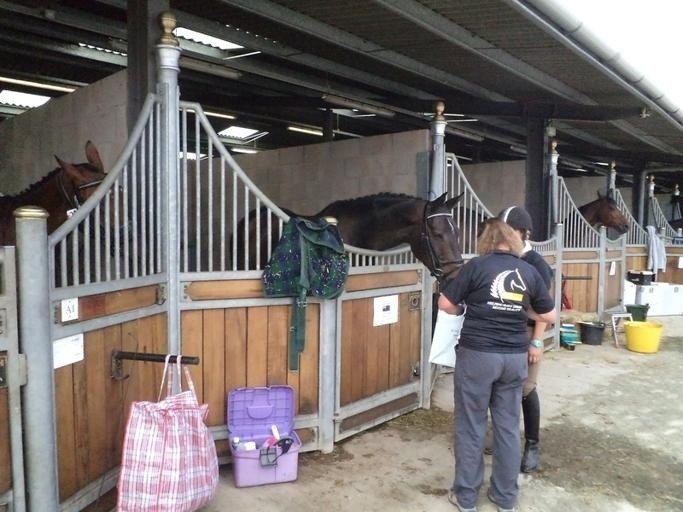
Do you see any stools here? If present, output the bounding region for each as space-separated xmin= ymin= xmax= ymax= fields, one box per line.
xmin=602 ymin=309 xmax=634 ymax=349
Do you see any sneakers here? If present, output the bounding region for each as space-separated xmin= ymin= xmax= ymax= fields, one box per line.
xmin=448 ymin=486 xmax=477 ymax=512
xmin=487 ymin=486 xmax=514 ymax=512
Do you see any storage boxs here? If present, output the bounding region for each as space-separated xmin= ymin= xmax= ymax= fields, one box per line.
xmin=627 ymin=269 xmax=655 ymax=286
xmin=226 ymin=383 xmax=303 ymax=489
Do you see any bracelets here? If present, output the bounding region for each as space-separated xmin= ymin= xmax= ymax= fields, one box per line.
xmin=529 ymin=339 xmax=542 ymax=347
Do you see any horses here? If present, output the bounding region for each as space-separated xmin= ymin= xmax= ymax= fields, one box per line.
xmin=0 ymin=138 xmax=146 ymax=266
xmin=560 ymin=186 xmax=631 ymax=250
xmin=450 ymin=206 xmax=489 ymax=254
xmin=229 ymin=187 xmax=468 ymax=288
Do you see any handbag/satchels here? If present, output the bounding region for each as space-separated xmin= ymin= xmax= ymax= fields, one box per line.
xmin=111 ymin=354 xmax=220 ymax=512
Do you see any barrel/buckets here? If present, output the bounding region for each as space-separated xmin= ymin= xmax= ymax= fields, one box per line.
xmin=580 ymin=321 xmax=606 ymax=345
xmin=625 ymin=321 xmax=664 ymax=353
xmin=626 ymin=305 xmax=649 ymax=321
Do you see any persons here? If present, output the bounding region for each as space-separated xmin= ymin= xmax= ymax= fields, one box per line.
xmin=482 ymin=204 xmax=555 ymax=476
xmin=436 ymin=216 xmax=559 ymax=511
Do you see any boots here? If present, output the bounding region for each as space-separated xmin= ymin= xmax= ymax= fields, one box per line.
xmin=519 ymin=387 xmax=539 ymax=472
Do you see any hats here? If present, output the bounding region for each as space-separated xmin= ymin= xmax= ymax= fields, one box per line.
xmin=498 ymin=205 xmax=533 ymax=232
